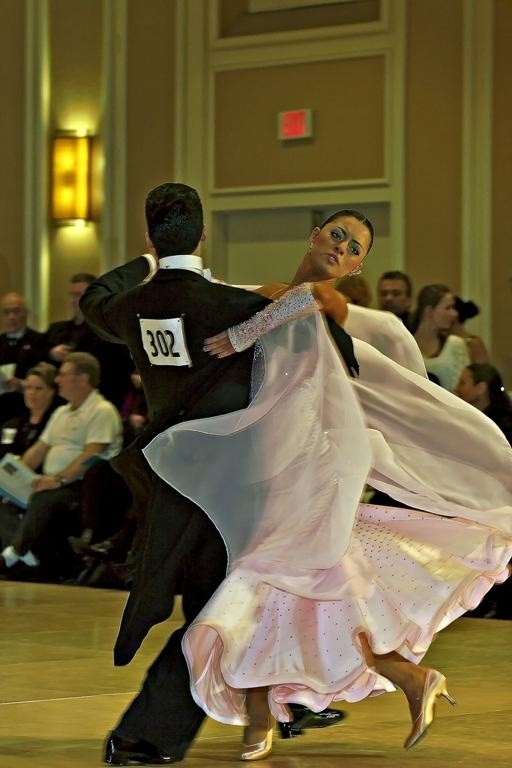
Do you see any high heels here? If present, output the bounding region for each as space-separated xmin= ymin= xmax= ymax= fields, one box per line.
xmin=404 ymin=668 xmax=455 ymax=750
xmin=240 ymin=718 xmax=273 ymax=760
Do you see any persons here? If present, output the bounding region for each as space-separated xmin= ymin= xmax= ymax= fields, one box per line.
xmin=77 ymin=182 xmax=361 ymax=765
xmin=143 ymin=209 xmax=511 ymax=762
xmin=340 ymin=271 xmax=512 ymax=621
xmin=1 ymin=276 xmax=150 ymax=586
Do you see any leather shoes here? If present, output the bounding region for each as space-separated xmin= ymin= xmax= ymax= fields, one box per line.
xmin=277 ymin=707 xmax=344 ymax=739
xmin=105 ymin=733 xmax=183 ymax=764
xmin=77 ymin=541 xmax=127 ymax=563
xmin=0 ymin=555 xmax=41 ymax=579
